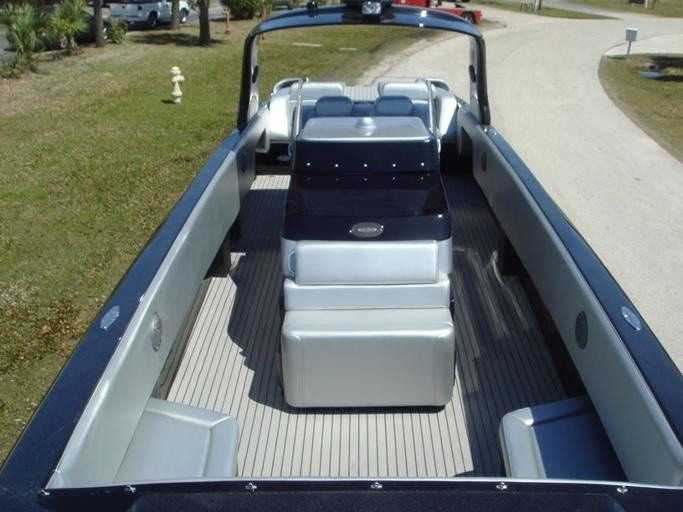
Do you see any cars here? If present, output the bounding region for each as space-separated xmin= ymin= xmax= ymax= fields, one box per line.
xmin=395 ymin=1 xmax=481 ymax=25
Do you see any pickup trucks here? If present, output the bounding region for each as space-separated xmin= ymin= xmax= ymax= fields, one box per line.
xmin=108 ymin=1 xmax=190 ymax=28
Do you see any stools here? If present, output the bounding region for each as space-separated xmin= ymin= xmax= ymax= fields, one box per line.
xmin=114 ymin=399 xmax=237 ymax=487
xmin=499 ymin=398 xmax=628 ymax=481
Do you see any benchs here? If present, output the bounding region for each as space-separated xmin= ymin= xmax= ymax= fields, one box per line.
xmin=288 ymin=96 xmax=438 ymax=157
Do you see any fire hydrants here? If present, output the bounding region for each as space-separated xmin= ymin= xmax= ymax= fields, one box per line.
xmin=169 ymin=67 xmax=184 ymax=104
